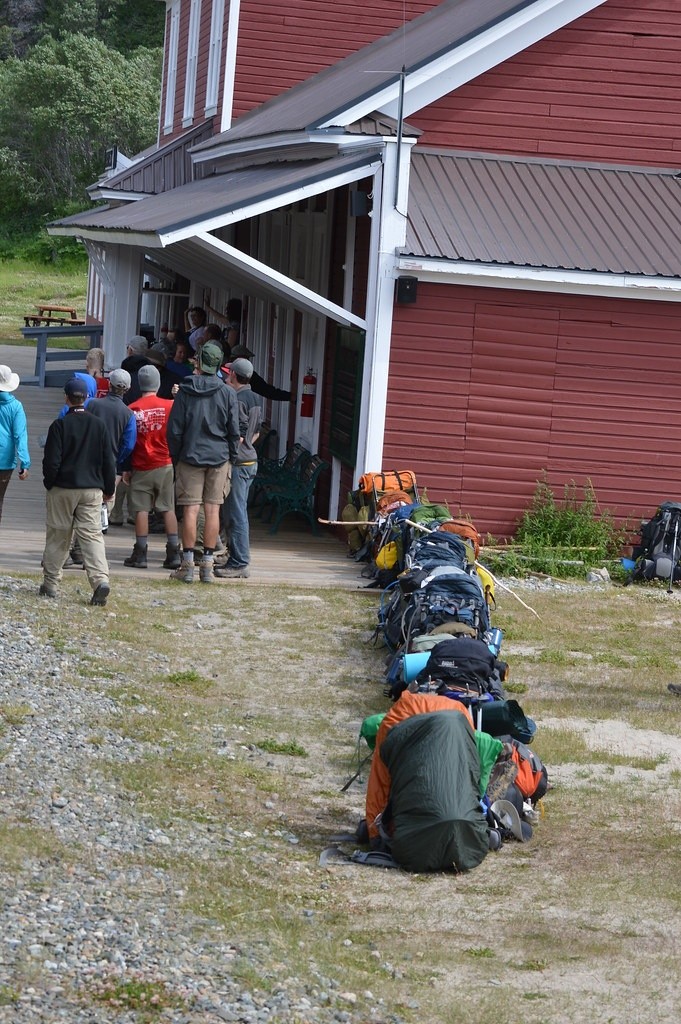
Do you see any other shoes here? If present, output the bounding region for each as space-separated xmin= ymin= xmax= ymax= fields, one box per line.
xmin=102 ymin=530 xmax=107 ymax=533
xmin=70 ymin=548 xmax=83 ymax=564
xmin=127 ymin=518 xmax=136 ymax=525
xmin=108 ymin=518 xmax=123 ymax=526
xmin=39 ymin=585 xmax=58 ymax=598
xmin=91 ymin=582 xmax=110 ymax=607
xmin=212 ymin=547 xmax=231 ymax=563
xmin=215 ymin=565 xmax=250 ymax=578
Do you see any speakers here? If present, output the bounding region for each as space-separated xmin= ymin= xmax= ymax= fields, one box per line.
xmin=396 ymin=275 xmax=418 ymax=303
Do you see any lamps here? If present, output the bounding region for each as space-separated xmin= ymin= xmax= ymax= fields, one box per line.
xmin=104 ymin=147 xmax=134 ymax=177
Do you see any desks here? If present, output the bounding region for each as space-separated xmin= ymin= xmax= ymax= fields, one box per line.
xmin=34 ymin=305 xmax=79 ymax=327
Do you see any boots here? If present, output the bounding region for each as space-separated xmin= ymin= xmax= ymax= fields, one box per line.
xmin=124 ymin=543 xmax=148 ymax=568
xmin=199 ymin=560 xmax=215 ymax=582
xmin=170 ymin=560 xmax=195 ymax=584
xmin=163 ymin=545 xmax=181 ymax=570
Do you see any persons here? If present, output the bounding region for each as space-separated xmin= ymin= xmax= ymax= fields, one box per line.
xmin=36 ymin=291 xmax=298 ymax=567
xmin=38 ymin=377 xmax=116 ymax=606
xmin=213 ymin=357 xmax=263 ymax=579
xmin=0 ymin=364 xmax=31 ymax=524
xmin=166 ymin=339 xmax=241 ymax=585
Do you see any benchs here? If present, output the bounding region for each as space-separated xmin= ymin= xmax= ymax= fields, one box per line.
xmin=24 ymin=315 xmax=85 ymax=326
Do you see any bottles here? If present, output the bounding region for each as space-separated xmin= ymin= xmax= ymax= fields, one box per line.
xmin=491 ymin=627 xmax=503 ymax=650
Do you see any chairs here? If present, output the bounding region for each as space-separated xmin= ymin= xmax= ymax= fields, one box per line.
xmin=254 ymin=440 xmax=328 ymax=536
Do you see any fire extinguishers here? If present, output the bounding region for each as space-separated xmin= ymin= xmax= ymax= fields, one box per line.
xmin=300 ymin=367 xmax=317 ymax=418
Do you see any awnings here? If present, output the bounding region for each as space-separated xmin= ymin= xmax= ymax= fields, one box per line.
xmin=40 ymin=149 xmax=387 ymax=333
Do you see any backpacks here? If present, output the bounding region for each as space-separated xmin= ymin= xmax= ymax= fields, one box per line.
xmin=625 ymin=501 xmax=681 ymax=583
xmin=355 ymin=471 xmax=548 ymax=874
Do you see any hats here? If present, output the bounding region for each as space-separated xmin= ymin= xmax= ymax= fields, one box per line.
xmin=231 ymin=345 xmax=256 ymax=360
xmin=0 ymin=364 xmax=20 ymax=393
xmin=142 ymin=350 xmax=166 ymax=365
xmin=197 ymin=344 xmax=224 ymax=374
xmin=126 ymin=336 xmax=148 ymax=350
xmin=138 ymin=365 xmax=161 ymax=389
xmin=63 ymin=378 xmax=87 ymax=397
xmin=225 ymin=358 xmax=253 ymax=379
xmin=108 ymin=368 xmax=131 ymax=390
